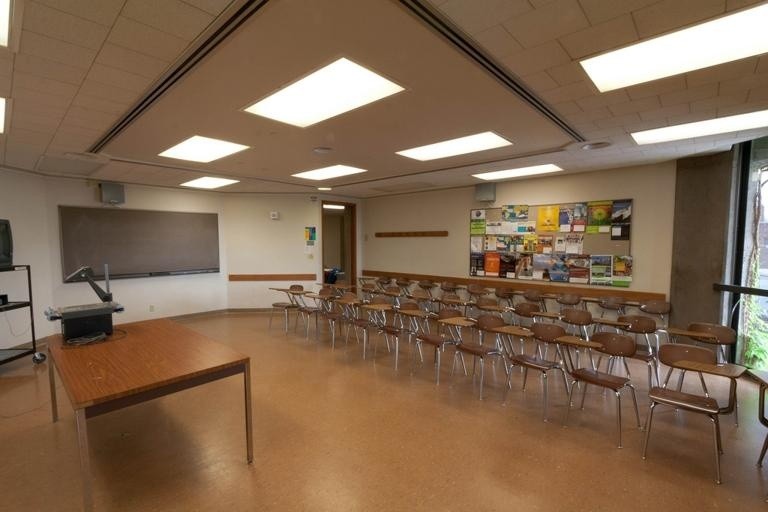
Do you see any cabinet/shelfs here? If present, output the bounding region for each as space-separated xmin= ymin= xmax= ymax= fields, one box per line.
xmin=0 ymin=261 xmax=39 ymax=371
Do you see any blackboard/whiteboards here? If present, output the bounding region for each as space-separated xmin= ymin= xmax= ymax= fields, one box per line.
xmin=57 ymin=204 xmax=220 ymax=284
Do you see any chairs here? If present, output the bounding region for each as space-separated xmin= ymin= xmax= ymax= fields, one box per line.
xmin=263 ymin=275 xmax=767 ymax=484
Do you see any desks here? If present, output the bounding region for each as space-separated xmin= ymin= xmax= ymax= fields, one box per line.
xmin=45 ymin=315 xmax=254 ymax=512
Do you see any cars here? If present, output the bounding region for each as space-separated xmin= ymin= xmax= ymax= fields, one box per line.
xmin=756 ymin=268 xmax=767 ymax=320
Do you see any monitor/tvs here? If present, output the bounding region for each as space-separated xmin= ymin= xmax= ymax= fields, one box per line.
xmin=0 ymin=219 xmax=13 ymax=270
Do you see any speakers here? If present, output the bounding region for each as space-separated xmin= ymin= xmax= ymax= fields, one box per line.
xmin=474 ymin=182 xmax=496 ymax=202
xmin=101 ymin=182 xmax=125 ymax=204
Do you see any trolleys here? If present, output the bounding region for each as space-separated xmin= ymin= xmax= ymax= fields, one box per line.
xmin=0 ymin=265 xmax=46 ymax=367
